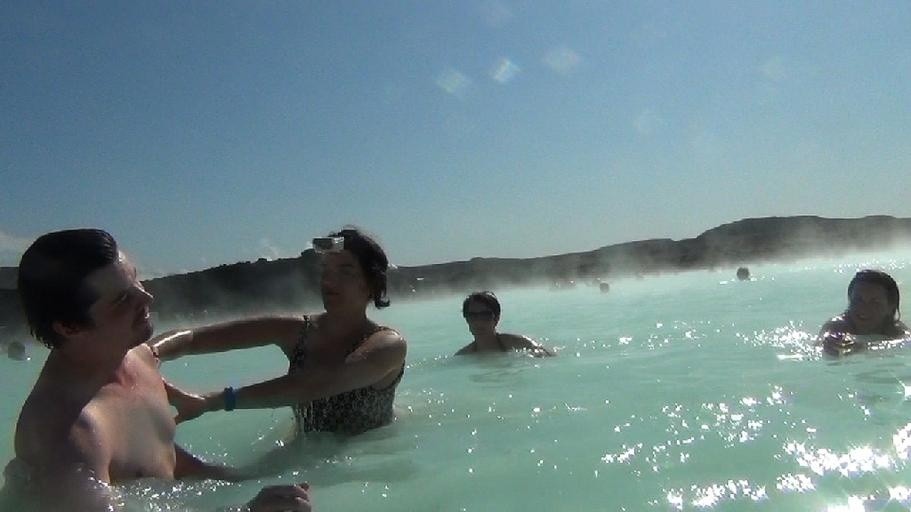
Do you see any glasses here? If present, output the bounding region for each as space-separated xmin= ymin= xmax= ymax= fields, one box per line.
xmin=312 ymin=236 xmax=345 ymax=254
xmin=465 ymin=310 xmax=494 ymax=321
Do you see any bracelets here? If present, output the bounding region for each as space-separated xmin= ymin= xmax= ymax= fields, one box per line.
xmin=224 ymin=386 xmax=233 ymax=412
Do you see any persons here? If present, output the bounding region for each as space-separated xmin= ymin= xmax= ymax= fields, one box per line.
xmin=600 ymin=283 xmax=609 ymax=294
xmin=737 ymin=268 xmax=751 ymax=283
xmin=454 ymin=292 xmax=552 ymax=364
xmin=814 ymin=269 xmax=910 ymax=357
xmin=152 ymin=230 xmax=407 ymax=434
xmin=14 ymin=229 xmax=310 ymax=512
